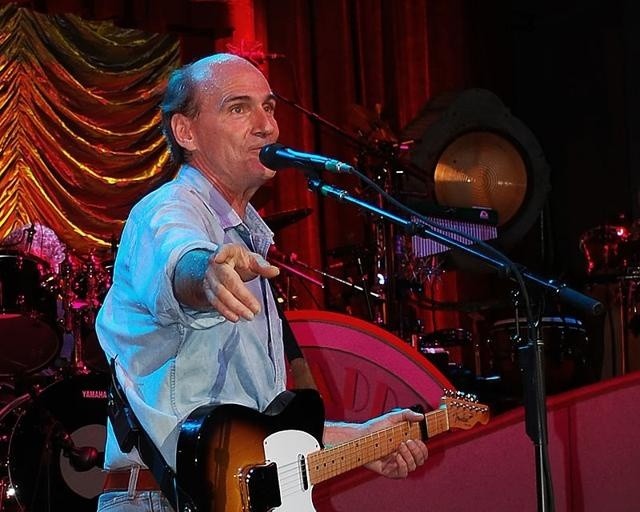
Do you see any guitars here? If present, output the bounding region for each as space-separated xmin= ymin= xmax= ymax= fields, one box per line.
xmin=175 ymin=388 xmax=490 ymax=512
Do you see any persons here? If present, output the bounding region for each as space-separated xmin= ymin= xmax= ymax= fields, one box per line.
xmin=92 ymin=52 xmax=429 ymax=511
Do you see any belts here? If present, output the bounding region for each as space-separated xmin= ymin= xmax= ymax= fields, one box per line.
xmin=100 ymin=469 xmax=163 ymax=492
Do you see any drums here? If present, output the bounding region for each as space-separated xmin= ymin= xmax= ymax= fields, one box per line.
xmin=0 ymin=375 xmax=107 ymax=511
xmin=582 ymin=224 xmax=639 ymax=281
xmin=0 ymin=249 xmax=64 ymax=377
xmin=485 ymin=317 xmax=591 ymax=417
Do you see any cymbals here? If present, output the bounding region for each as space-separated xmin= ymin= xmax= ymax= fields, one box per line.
xmin=260 ymin=208 xmax=312 ymax=231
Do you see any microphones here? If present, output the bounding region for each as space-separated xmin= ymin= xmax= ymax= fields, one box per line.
xmin=307 ymin=176 xmax=349 ymax=203
xmin=24 ymin=224 xmax=36 ymax=256
xmin=259 ymin=142 xmax=355 ymax=175
